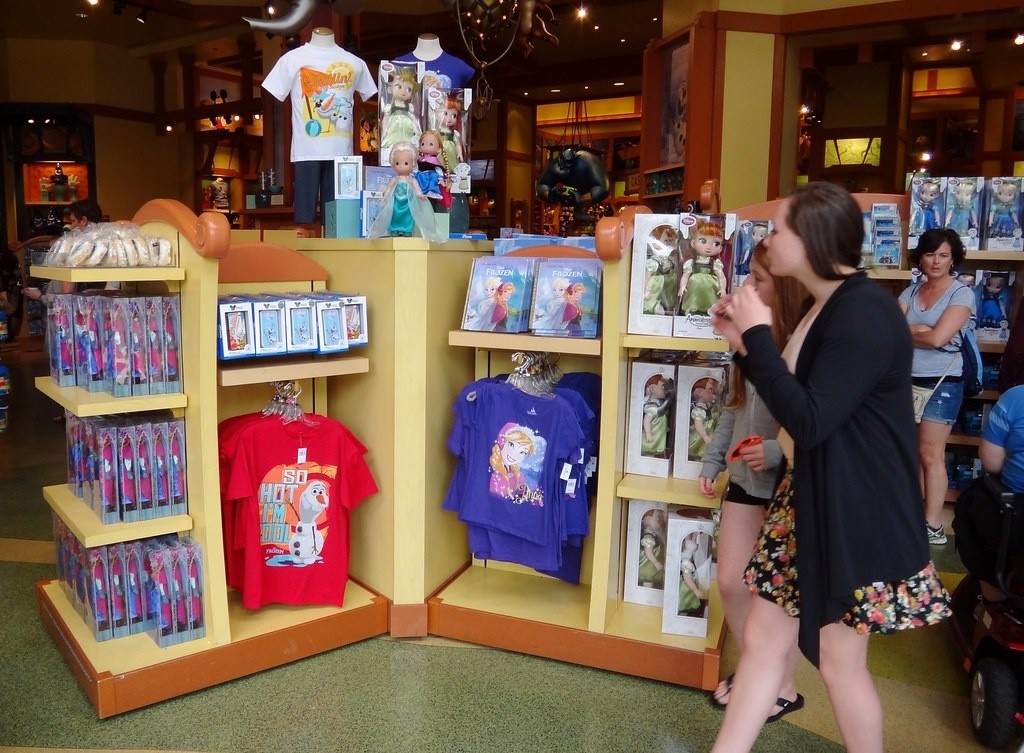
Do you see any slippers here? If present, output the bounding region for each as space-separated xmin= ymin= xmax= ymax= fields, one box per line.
xmin=709 ymin=673 xmax=735 ymax=711
xmin=765 ymin=693 xmax=804 ymax=723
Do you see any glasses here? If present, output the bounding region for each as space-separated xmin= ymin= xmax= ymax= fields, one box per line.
xmin=729 ymin=435 xmax=766 ymax=462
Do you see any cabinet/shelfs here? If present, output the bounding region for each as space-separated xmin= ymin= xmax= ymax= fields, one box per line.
xmin=31 ymin=198 xmax=729 ymax=719
xmin=702 ymin=179 xmax=1018 ymax=502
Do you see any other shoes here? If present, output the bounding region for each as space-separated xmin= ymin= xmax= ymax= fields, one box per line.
xmin=53 ymin=415 xmax=65 ymax=422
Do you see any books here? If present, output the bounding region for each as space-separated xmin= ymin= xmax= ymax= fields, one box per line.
xmin=461 ymin=255 xmax=603 ymax=339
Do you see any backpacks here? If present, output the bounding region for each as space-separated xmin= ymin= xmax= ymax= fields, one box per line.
xmin=912 ymin=283 xmax=983 ymax=399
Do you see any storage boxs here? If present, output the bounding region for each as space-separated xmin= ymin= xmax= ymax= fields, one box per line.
xmin=907 ymin=177 xmax=1024 ymax=342
xmin=378 ymin=60 xmax=472 ymax=166
xmin=620 ymin=211 xmax=769 ymax=636
xmin=45 ymin=294 xmax=206 ymax=647
xmin=324 ymin=166 xmax=396 ymax=237
xmin=209 ymin=291 xmax=369 ymax=360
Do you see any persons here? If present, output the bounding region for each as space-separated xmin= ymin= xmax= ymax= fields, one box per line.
xmin=640 ymin=215 xmax=770 ymax=617
xmin=62 ymin=198 xmax=109 ymax=297
xmin=710 ymin=180 xmax=956 ymax=753
xmin=909 ymin=176 xmax=1022 ymax=328
xmin=896 ymin=229 xmax=975 ymax=544
xmin=364 ymin=71 xmax=466 ymax=243
xmin=50 ymin=291 xmax=201 ymax=633
xmin=697 ymin=236 xmax=814 ymax=724
xmin=950 ymin=342 xmax=1024 ymax=613
xmin=21 ymin=217 xmax=66 ymax=423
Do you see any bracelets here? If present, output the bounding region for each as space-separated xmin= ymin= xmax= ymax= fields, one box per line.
xmin=37 ymin=293 xmax=42 ymax=301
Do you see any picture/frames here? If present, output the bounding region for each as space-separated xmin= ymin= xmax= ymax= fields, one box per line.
xmin=334 ymin=154 xmax=364 ymax=198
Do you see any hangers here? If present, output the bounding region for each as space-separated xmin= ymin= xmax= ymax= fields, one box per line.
xmin=505 ymin=351 xmax=564 ymax=400
xmin=261 ymin=381 xmax=314 ymax=425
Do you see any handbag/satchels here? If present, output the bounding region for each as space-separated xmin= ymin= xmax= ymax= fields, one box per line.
xmin=952 ymin=473 xmax=1024 ymax=602
xmin=912 ymin=385 xmax=933 ymax=423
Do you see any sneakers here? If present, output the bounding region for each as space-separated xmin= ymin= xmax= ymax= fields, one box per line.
xmin=926 ymin=520 xmax=947 ymax=544
